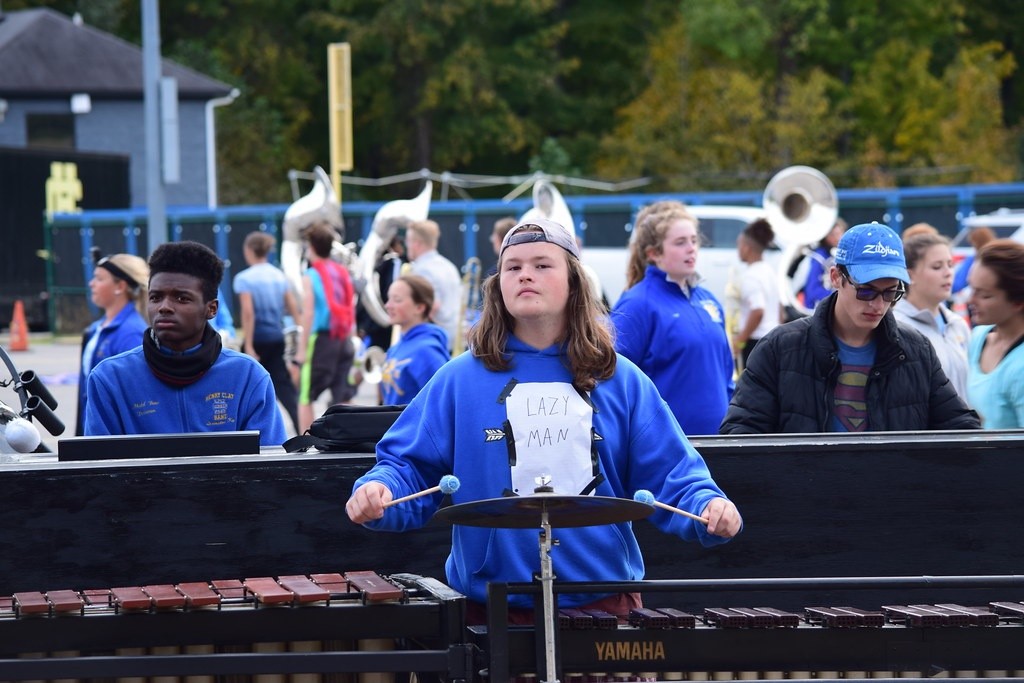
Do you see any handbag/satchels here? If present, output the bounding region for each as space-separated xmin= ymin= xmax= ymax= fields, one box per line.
xmin=282 ymin=404 xmax=410 ymax=454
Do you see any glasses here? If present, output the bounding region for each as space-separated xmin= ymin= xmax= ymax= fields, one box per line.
xmin=839 ymin=269 xmax=906 ymax=302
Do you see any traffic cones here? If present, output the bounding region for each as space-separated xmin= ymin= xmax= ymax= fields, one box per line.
xmin=9 ymin=301 xmax=30 ymax=351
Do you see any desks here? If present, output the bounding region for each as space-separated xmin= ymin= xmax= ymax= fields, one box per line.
xmin=0 ymin=428 xmax=1024 ymax=608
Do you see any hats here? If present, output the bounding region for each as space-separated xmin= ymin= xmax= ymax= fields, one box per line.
xmin=834 ymin=221 xmax=910 ymax=285
xmin=500 ymin=219 xmax=580 ymax=261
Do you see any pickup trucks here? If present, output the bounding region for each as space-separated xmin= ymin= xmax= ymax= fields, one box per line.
xmin=575 ymin=205 xmax=807 ymax=342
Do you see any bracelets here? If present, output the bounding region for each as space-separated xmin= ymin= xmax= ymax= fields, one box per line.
xmin=734 ymin=338 xmax=746 ymax=348
xmin=292 ymin=360 xmax=301 ymax=366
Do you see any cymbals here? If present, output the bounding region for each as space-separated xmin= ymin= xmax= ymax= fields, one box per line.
xmin=432 ymin=495 xmax=654 ymax=530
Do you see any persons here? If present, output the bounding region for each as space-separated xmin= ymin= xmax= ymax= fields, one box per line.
xmin=804 ymin=219 xmax=848 ymax=310
xmin=608 ymin=201 xmax=735 ymax=435
xmin=288 ymin=228 xmax=356 ymax=434
xmin=953 ymin=227 xmax=1024 ymax=429
xmin=84 ymin=241 xmax=288 ymax=446
xmin=892 ymin=223 xmax=970 ymax=400
xmin=380 ymin=276 xmax=451 ymax=406
xmin=718 ymin=220 xmax=982 ymax=434
xmin=732 ymin=219 xmax=785 ymax=371
xmin=234 ymin=231 xmax=297 ymax=428
xmin=404 ymin=218 xmax=463 ymax=350
xmin=346 ymin=217 xmax=744 ymax=623
xmin=80 ymin=253 xmax=149 ymax=427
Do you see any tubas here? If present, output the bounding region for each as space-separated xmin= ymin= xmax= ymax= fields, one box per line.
xmin=278 ymin=165 xmax=340 ymax=320
xmin=357 ymin=178 xmax=434 ymax=326
xmin=762 ymin=164 xmax=840 ymax=313
